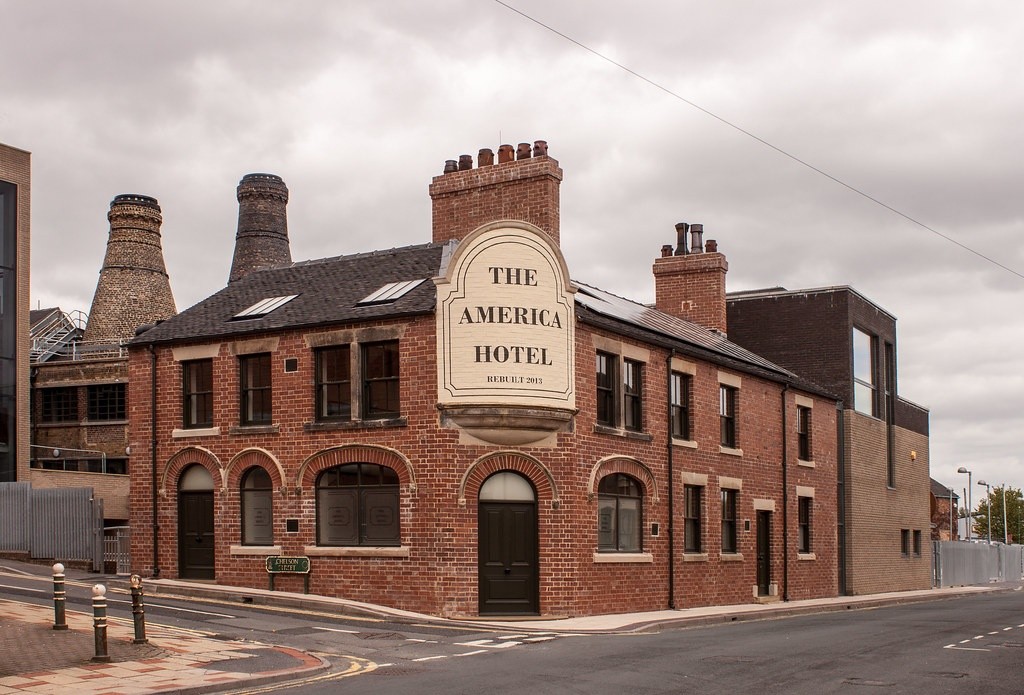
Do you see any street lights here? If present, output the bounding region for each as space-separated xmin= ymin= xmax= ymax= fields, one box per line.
xmin=978 ymin=480 xmax=991 ymax=544
xmin=946 ymin=487 xmax=954 ymax=542
xmin=958 ymin=467 xmax=972 ymax=543
